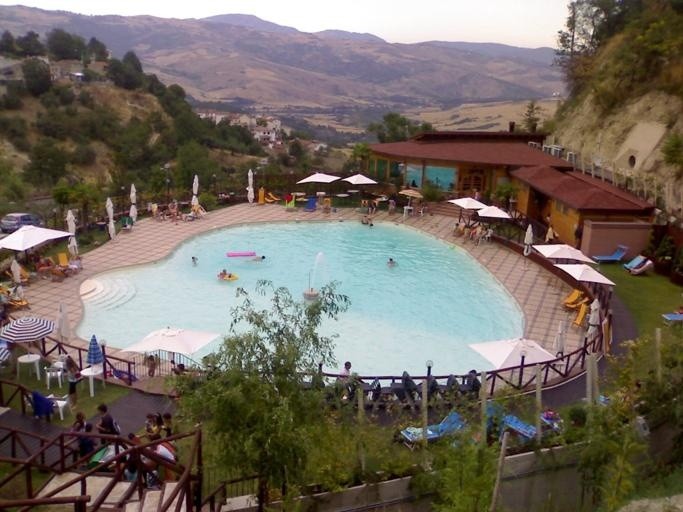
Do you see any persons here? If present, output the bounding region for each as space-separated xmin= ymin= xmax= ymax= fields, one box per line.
xmin=337 ymin=361 xmax=351 ymax=381
xmin=65 ymin=354 xmax=189 ymax=485
xmin=387 ymin=259 xmax=396 ymax=265
xmin=467 ymin=370 xmax=481 ymax=398
xmin=253 ymin=255 xmax=266 ymax=262
xmin=219 ymin=269 xmax=228 ymax=276
xmin=452 ymin=222 xmax=493 ymax=247
xmin=359 ymin=201 xmax=379 ymax=227
xmin=191 ymin=256 xmax=199 ymax=265
xmin=149 ymin=200 xmax=207 ymax=225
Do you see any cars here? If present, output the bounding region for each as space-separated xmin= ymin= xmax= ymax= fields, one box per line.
xmin=1 ymin=213 xmax=40 ymax=233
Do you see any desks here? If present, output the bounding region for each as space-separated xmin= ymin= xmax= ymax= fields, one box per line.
xmin=16 ymin=354 xmax=41 ymax=381
xmin=80 ymin=367 xmax=106 ymax=398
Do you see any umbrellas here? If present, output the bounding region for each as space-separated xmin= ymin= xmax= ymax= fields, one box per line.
xmin=66 ymin=209 xmax=78 ymax=257
xmin=191 ymin=175 xmax=199 ymax=206
xmin=588 ymin=299 xmax=600 ymax=354
xmin=448 ymin=197 xmax=488 ymax=223
xmin=0 ymin=348 xmax=11 ymax=364
xmin=11 ymin=260 xmax=23 ymax=302
xmin=524 ymin=223 xmax=533 ymax=268
xmin=105 ymin=197 xmax=115 ymax=238
xmin=119 ymin=326 xmax=219 ymax=355
xmin=553 ymin=322 xmax=564 ymax=353
xmin=128 ymin=183 xmax=137 ymax=222
xmin=0 ymin=316 xmax=55 ymax=359
xmin=477 ymin=206 xmax=512 ymax=229
xmin=532 ymin=244 xmax=596 ymax=263
xmin=246 ymin=169 xmax=255 ymax=203
xmin=399 ymin=189 xmax=423 ymax=206
xmin=0 ymin=225 xmax=75 ymax=264
xmin=296 ymin=173 xmax=342 ymax=184
xmin=470 ymin=337 xmax=557 ymax=369
xmin=553 ymin=263 xmax=616 ymax=285
xmin=87 ymin=334 xmax=104 ymax=365
xmin=340 ymin=174 xmax=378 ymax=185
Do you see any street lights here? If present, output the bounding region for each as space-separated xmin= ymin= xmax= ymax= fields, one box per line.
xmin=426 ymin=361 xmax=433 ymax=377
xmin=316 ymin=355 xmax=324 ymax=379
xmin=99 ymin=338 xmax=107 ymax=378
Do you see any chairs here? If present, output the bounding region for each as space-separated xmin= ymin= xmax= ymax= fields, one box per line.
xmin=662 ymin=314 xmax=683 ymax=328
xmin=624 ymin=254 xmax=647 ymax=272
xmin=399 ymin=406 xmax=467 ymax=453
xmin=265 ymin=192 xmax=331 ymax=213
xmin=0 ymin=253 xmax=78 ymax=311
xmin=592 ymin=245 xmax=627 ymax=265
xmin=561 ymin=289 xmax=592 ymax=329
xmin=26 ymin=361 xmax=73 ymax=422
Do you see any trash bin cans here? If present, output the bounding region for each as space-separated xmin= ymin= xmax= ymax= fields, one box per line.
xmin=96 ymin=222 xmax=106 ymax=231
xmin=361 ymin=200 xmax=368 ymax=213
xmin=412 ymin=198 xmax=422 ymax=216
